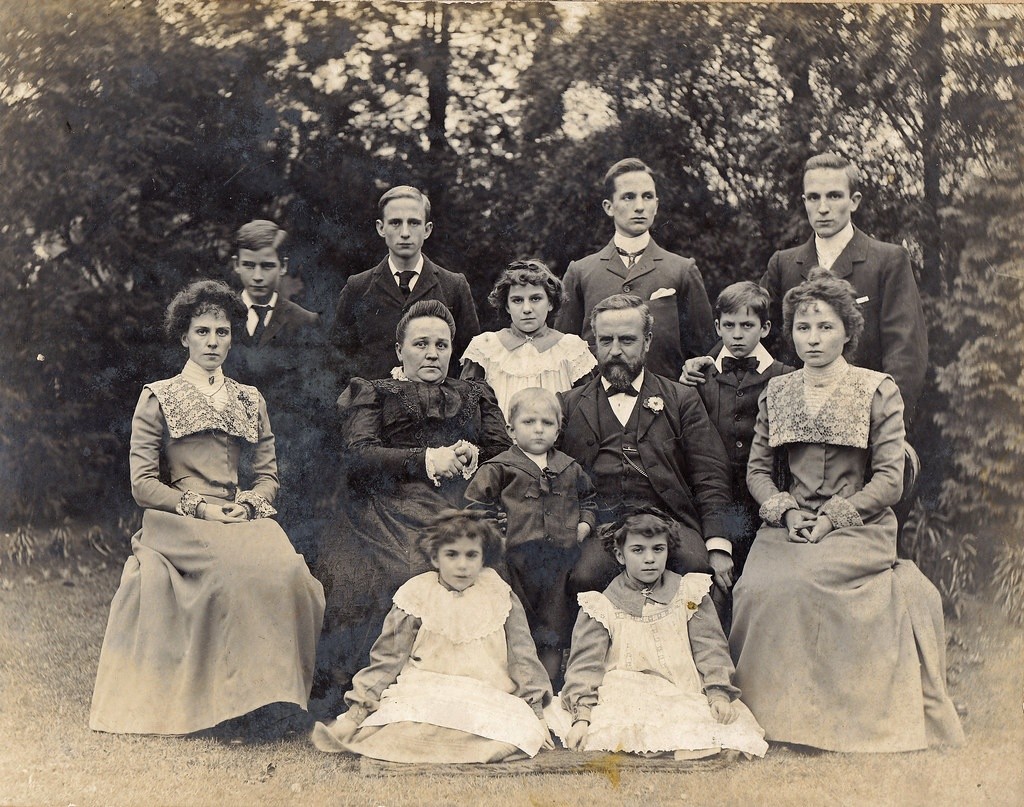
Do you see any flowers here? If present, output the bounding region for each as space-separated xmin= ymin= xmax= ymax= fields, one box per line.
xmin=642 ymin=396 xmax=664 ymax=415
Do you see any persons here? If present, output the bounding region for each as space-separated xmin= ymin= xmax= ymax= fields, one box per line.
xmin=458 ymin=258 xmax=601 ymax=442
xmin=676 ymin=277 xmax=796 ymax=577
xmin=330 ymin=294 xmax=512 ymax=676
xmin=324 ymin=185 xmax=483 ymax=383
xmin=556 ymin=512 xmax=770 ymax=764
xmin=310 ymin=502 xmax=555 ymax=767
xmin=675 ymin=154 xmax=927 ymax=444
xmin=545 ymin=294 xmax=736 ymax=629
xmin=552 ymin=154 xmax=719 ymax=385
xmin=84 ymin=279 xmax=326 ymax=735
xmin=198 ymin=219 xmax=324 ymax=593
xmin=466 ymin=389 xmax=598 ymax=708
xmin=714 ymin=273 xmax=966 ymax=757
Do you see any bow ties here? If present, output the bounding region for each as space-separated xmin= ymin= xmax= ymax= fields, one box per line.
xmin=605 ymin=369 xmax=643 ymax=398
xmin=722 ymin=356 xmax=760 ymax=375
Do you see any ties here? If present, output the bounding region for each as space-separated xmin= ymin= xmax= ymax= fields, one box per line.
xmin=250 ymin=304 xmax=272 ymax=345
xmin=395 ymin=270 xmax=417 ymax=300
xmin=617 ymin=246 xmax=647 ymax=268
